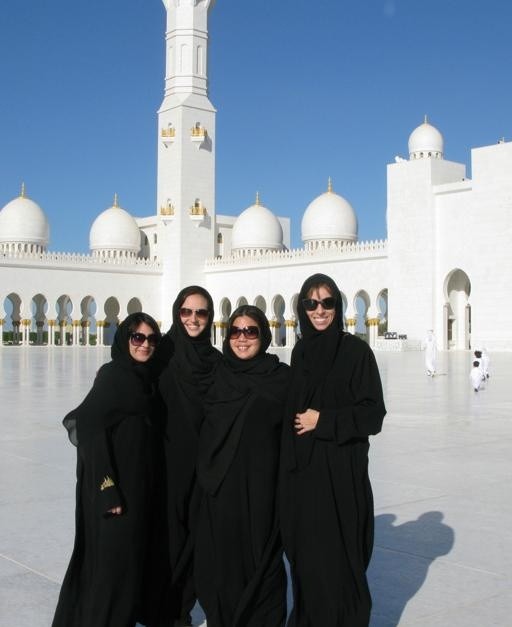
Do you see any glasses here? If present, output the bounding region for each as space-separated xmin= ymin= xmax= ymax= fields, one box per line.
xmin=131 ymin=332 xmax=159 ymax=347
xmin=228 ymin=325 xmax=257 ymax=339
xmin=180 ymin=308 xmax=210 ymax=319
xmin=301 ymin=297 xmax=335 ymax=311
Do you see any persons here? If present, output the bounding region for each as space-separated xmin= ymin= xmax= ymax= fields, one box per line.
xmin=54 ymin=312 xmax=162 ymax=626
xmin=284 ymin=272 xmax=385 ymax=626
xmin=151 ymin=285 xmax=223 ymax=626
xmin=423 ymin=327 xmax=492 ymax=391
xmin=195 ymin=305 xmax=292 ymax=625
